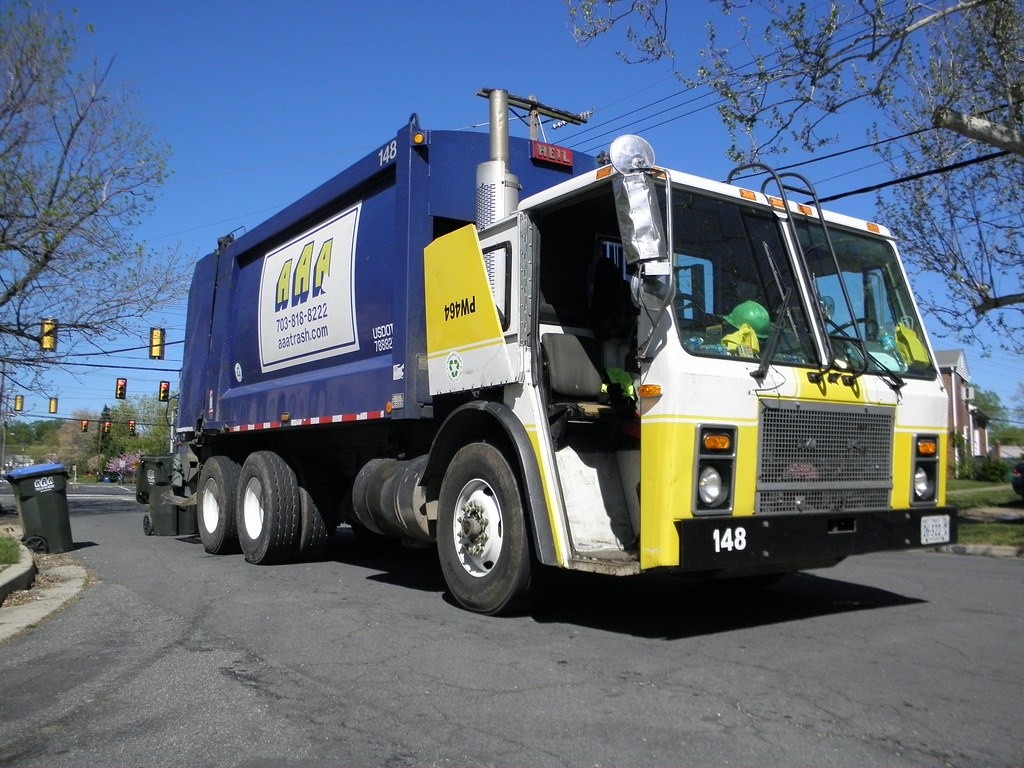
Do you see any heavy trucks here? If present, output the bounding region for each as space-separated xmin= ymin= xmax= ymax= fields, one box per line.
xmin=176 ymin=89 xmax=959 ymax=616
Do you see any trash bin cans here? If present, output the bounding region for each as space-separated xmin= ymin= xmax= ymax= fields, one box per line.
xmin=2 ymin=461 xmax=76 ymax=554
xmin=134 ymin=450 xmax=197 ymax=537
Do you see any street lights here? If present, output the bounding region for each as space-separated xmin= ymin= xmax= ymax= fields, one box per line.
xmin=22 ymin=448 xmax=24 ymax=466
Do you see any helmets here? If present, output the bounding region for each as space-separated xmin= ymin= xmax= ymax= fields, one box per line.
xmin=724 ymin=301 xmax=769 ymax=339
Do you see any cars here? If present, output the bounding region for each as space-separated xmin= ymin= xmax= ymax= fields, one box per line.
xmin=101 ymin=471 xmax=122 ymax=480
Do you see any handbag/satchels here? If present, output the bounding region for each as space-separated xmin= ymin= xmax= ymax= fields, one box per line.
xmin=895 ymin=316 xmax=930 ymax=371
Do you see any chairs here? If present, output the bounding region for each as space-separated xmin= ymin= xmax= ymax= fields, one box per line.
xmin=542 ymin=331 xmax=630 ymax=423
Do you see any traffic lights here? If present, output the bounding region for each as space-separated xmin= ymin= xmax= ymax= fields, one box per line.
xmin=81 ymin=421 xmax=88 ymax=432
xmin=159 ymin=381 xmax=170 ymax=402
xmin=129 ymin=420 xmax=135 ymax=432
xmin=115 ymin=378 xmax=126 ymax=399
xmin=105 ymin=422 xmax=110 ymax=433
